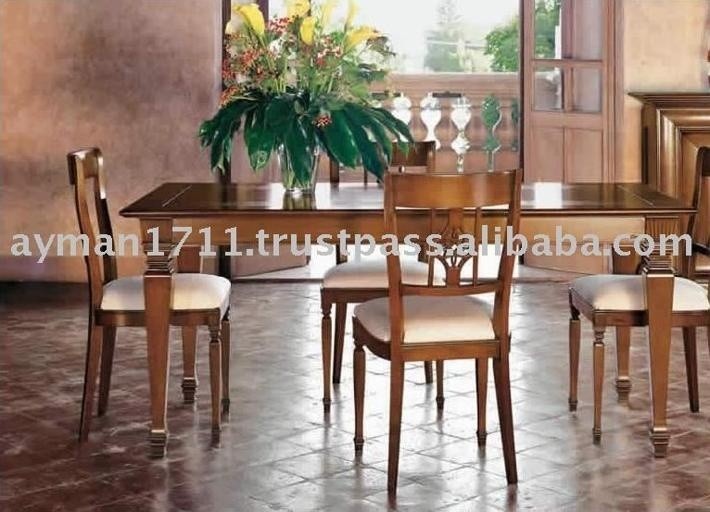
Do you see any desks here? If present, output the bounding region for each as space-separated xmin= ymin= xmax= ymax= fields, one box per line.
xmin=118 ymin=180 xmax=699 ymax=462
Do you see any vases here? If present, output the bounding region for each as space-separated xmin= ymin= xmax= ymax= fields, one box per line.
xmin=274 ymin=133 xmax=320 ymax=193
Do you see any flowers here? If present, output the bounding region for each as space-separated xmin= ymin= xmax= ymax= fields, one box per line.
xmin=198 ymin=0 xmax=418 ymax=189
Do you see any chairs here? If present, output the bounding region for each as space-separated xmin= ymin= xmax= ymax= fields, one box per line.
xmin=564 ymin=147 xmax=709 ymax=444
xmin=64 ymin=142 xmax=235 ymax=439
xmin=321 ymin=138 xmax=527 ymax=500
